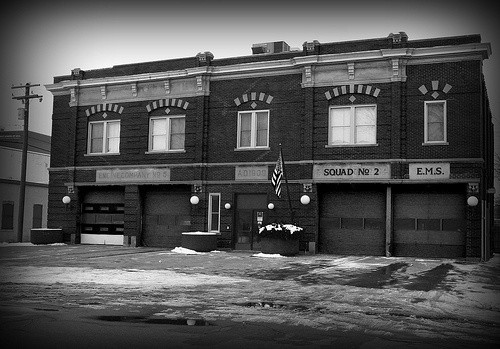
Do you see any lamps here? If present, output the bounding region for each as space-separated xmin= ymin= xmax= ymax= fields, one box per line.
xmin=62 ymin=195 xmax=75 ymax=210
xmin=225 ymin=202 xmax=232 ymax=216
xmin=300 ymin=194 xmax=315 ymax=205
xmin=190 ymin=194 xmax=201 ymax=211
xmin=467 ymin=195 xmax=479 ymax=210
xmin=268 ymin=202 xmax=276 ymax=216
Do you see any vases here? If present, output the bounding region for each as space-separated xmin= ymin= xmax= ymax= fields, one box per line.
xmin=259 ymin=232 xmax=301 ymax=253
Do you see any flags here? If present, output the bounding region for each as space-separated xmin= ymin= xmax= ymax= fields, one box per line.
xmin=271 ymin=151 xmax=284 ymax=199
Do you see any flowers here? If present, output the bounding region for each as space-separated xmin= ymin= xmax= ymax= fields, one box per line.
xmin=258 ymin=222 xmax=303 ymax=241
